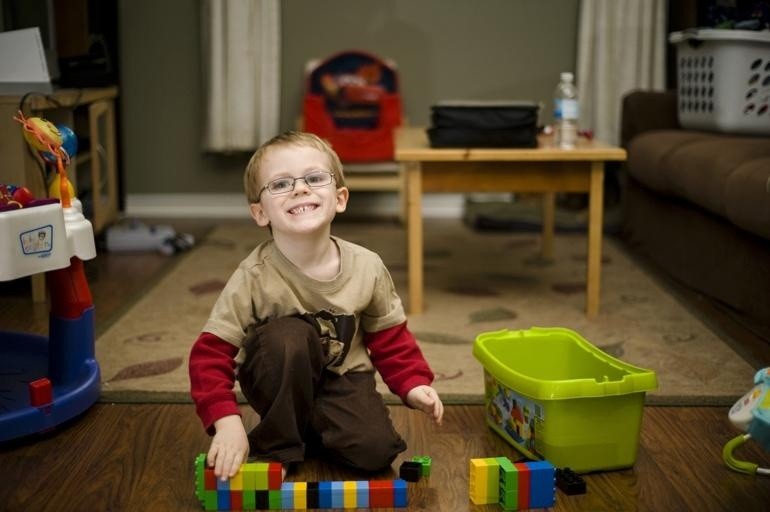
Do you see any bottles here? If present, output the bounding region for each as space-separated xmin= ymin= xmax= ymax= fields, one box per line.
xmin=553 ymin=70 xmax=580 ymax=148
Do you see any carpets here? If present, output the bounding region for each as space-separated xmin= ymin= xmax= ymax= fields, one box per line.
xmin=90 ymin=217 xmax=761 ymax=403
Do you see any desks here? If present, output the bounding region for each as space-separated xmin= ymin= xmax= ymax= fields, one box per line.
xmin=393 ymin=126 xmax=629 ymax=315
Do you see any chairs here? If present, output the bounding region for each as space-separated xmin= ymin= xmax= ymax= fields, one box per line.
xmin=297 ymin=57 xmax=411 ymax=228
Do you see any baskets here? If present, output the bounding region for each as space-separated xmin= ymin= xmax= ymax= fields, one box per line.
xmin=666 ymin=25 xmax=770 ymax=135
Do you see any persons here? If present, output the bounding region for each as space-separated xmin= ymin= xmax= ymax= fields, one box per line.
xmin=189 ymin=129 xmax=445 ymax=483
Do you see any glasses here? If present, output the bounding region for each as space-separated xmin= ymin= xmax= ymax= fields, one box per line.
xmin=255 ymin=170 xmax=340 ymax=204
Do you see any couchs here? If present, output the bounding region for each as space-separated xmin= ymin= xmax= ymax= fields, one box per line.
xmin=621 ymin=90 xmax=770 ymax=348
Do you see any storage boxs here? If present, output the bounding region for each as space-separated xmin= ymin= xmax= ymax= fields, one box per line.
xmin=472 ymin=326 xmax=657 ymax=475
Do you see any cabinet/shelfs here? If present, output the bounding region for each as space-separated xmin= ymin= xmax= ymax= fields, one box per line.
xmin=0 ymin=87 xmax=119 ymax=304
